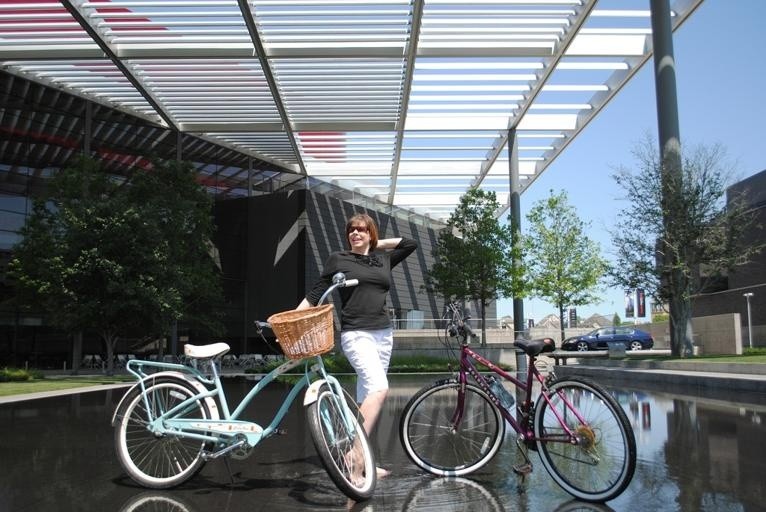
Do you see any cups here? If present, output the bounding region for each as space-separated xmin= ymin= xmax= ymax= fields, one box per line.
xmin=484 ymin=374 xmax=515 ymax=410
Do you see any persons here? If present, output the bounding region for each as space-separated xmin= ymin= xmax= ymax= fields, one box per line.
xmin=274 ymin=213 xmax=419 ymax=489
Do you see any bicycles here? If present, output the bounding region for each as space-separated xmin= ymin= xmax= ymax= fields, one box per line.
xmin=399 ymin=297 xmax=637 ymax=502
xmin=110 ymin=271 xmax=377 ymax=502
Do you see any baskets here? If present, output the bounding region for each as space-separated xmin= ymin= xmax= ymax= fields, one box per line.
xmin=266 ymin=303 xmax=335 ymax=360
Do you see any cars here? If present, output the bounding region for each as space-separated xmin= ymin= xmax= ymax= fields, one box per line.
xmin=562 ymin=326 xmax=654 ymax=350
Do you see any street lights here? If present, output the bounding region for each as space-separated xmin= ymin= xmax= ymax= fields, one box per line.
xmin=742 ymin=291 xmax=755 ymax=350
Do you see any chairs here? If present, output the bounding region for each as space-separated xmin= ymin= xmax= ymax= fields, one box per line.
xmin=82 ymin=353 xmax=284 ymax=377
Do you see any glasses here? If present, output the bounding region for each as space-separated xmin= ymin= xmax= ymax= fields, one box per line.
xmin=349 ymin=226 xmax=367 ymax=233
